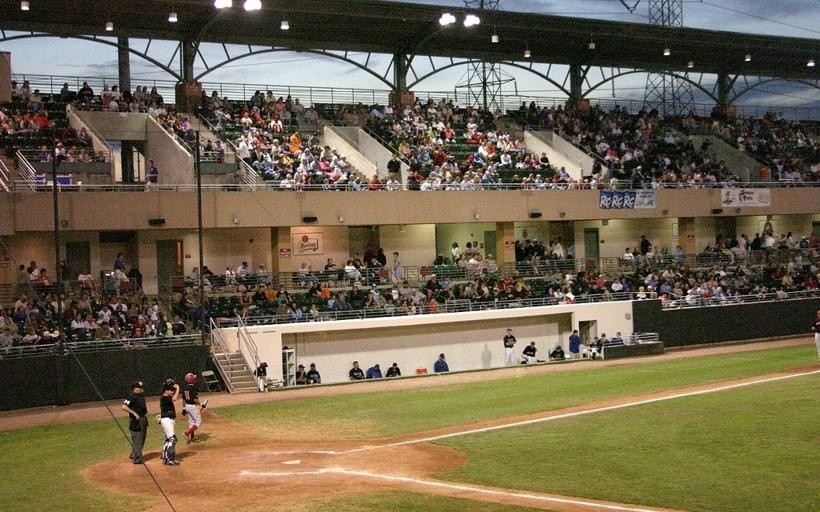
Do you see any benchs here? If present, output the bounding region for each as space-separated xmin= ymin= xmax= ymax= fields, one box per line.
xmin=171 ymin=274 xmax=270 ymax=294
xmin=421 ymin=264 xmax=501 ymax=282
xmin=0 ymin=282 xmax=64 ymax=303
xmin=515 ymin=259 xmax=599 ymax=277
xmin=695 ymin=249 xmax=788 ymax=266
xmin=619 ymin=254 xmax=685 ymax=271
xmin=292 ymin=268 xmax=382 ymax=291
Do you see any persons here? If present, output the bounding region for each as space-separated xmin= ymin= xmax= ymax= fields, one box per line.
xmin=1 ymin=232 xmax=820 ymax=358
xmin=121 ymin=381 xmax=149 ymax=464
xmin=296 ymin=363 xmax=321 ymax=385
xmin=349 ymin=361 xmax=401 ymax=381
xmin=1 ymin=80 xmax=820 ymax=192
xmin=433 ymin=353 xmax=450 ymax=372
xmin=181 ymin=373 xmax=206 ymax=444
xmin=503 ymin=328 xmax=537 ymax=365
xmin=254 ymin=362 xmax=269 ymax=392
xmin=160 ymin=379 xmax=181 ymax=466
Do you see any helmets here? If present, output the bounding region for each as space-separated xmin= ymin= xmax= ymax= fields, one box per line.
xmin=185 ymin=373 xmax=197 ymax=385
xmin=162 ymin=378 xmax=174 ymax=389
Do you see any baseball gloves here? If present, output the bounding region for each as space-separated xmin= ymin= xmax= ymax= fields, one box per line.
xmin=155 ymin=414 xmax=161 ymax=424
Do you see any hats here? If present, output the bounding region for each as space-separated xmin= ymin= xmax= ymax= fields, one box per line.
xmin=132 ymin=381 xmax=144 ymax=390
xmin=299 ymin=365 xmax=305 ymax=368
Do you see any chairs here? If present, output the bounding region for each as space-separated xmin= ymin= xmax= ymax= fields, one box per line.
xmin=416 ymin=368 xmax=427 ymax=374
xmin=198 ymin=100 xmax=559 ymax=190
xmin=208 ymin=290 xmax=400 ymax=327
xmin=0 ymin=95 xmax=88 ymax=159
xmin=29 ymin=174 xmax=47 ymax=184
xmin=522 ymin=279 xmax=610 ymax=301
xmin=201 ymin=370 xmax=221 ymax=392
xmin=56 ymin=175 xmax=82 ymax=191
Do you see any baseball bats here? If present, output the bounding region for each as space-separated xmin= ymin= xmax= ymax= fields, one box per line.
xmin=199 ymin=400 xmax=208 ymax=412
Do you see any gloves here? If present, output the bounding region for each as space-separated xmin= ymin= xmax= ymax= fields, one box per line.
xmin=182 ymin=409 xmax=187 ymax=417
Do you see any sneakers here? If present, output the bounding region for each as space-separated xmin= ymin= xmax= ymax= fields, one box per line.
xmin=182 ymin=431 xmax=190 ymax=442
xmin=168 ymin=460 xmax=180 ymax=466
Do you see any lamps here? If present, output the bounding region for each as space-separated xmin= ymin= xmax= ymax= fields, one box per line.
xmin=588 ymin=33 xmax=594 ymax=50
xmin=688 ymin=54 xmax=694 ymax=68
xmin=664 ymin=42 xmax=669 ymax=55
xmin=492 ymin=27 xmax=498 ymax=43
xmin=807 ymin=59 xmax=814 ymax=67
xmin=745 ymin=48 xmax=751 ymax=61
xmin=524 ymin=42 xmax=530 ymax=58
